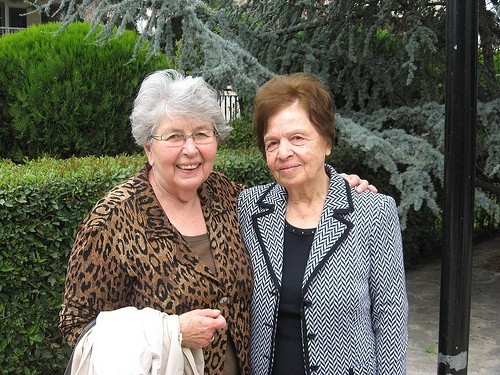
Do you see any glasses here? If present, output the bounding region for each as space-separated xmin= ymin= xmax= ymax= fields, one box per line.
xmin=147 ymin=129 xmax=218 ymax=147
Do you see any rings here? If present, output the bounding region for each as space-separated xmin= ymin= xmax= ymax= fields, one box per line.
xmin=210 ymin=335 xmax=215 ymax=343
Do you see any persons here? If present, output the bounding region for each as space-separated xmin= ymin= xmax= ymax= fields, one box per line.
xmin=238 ymin=72 xmax=409 ymax=375
xmin=58 ymin=69 xmax=378 ymax=375
